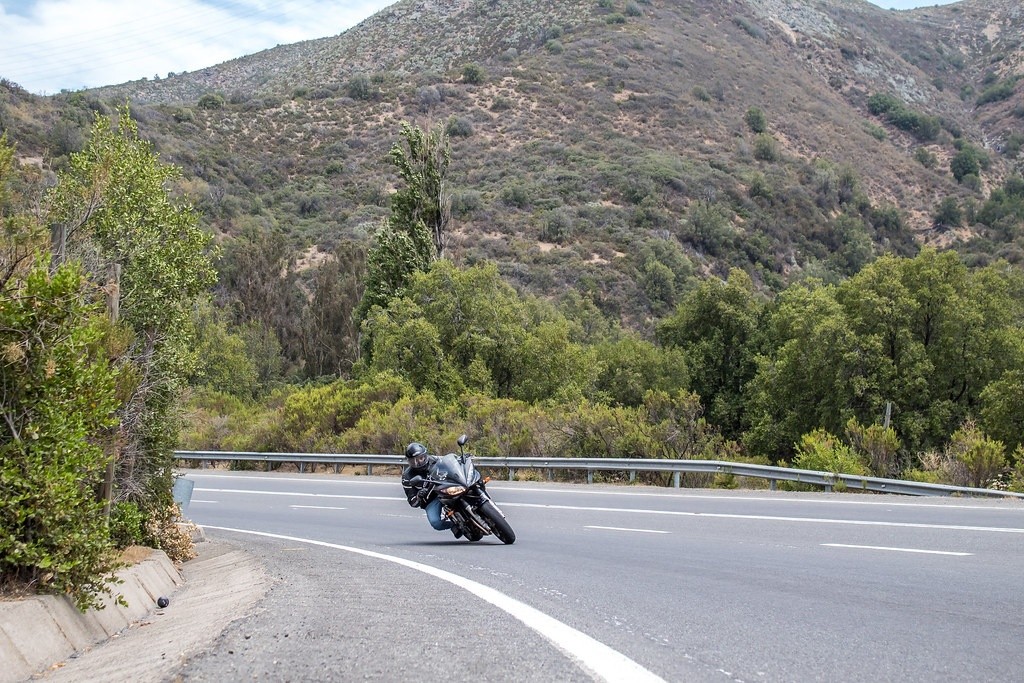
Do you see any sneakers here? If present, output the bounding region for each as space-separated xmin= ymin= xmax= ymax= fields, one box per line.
xmin=451 ymin=523 xmax=462 ymax=539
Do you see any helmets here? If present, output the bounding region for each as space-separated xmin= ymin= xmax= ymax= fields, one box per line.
xmin=405 ymin=443 xmax=428 ymax=471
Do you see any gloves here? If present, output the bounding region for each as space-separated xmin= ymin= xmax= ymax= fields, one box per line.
xmin=418 ymin=488 xmax=429 ymax=499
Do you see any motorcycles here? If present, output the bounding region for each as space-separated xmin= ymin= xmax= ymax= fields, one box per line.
xmin=409 ymin=436 xmax=516 ymax=545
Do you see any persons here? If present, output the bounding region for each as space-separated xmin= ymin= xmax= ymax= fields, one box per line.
xmin=401 ymin=442 xmax=474 ymax=539
